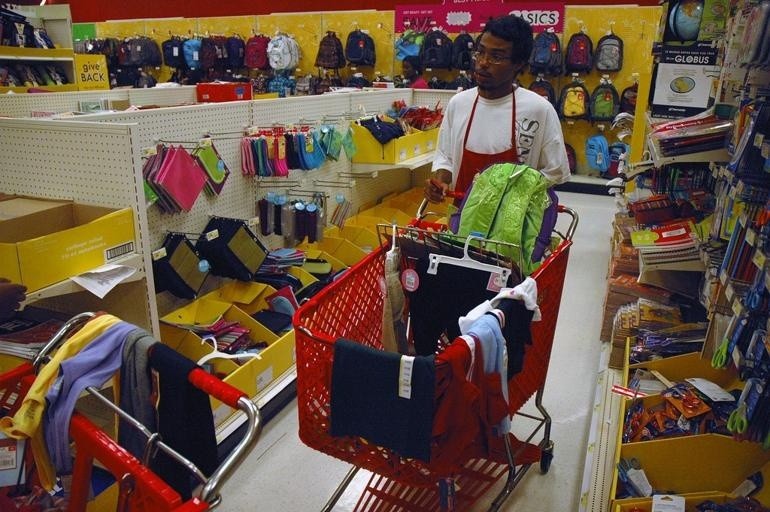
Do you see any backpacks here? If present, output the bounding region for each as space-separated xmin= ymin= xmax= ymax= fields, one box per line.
xmin=419 ymin=31 xmax=476 ymax=69
xmin=528 ymin=30 xmax=623 ymax=122
xmin=563 ymin=143 xmax=577 ymax=173
xmin=85 ymin=36 xmax=162 ymax=87
xmin=160 ymin=33 xmax=298 ymax=97
xmin=296 ymin=31 xmax=376 ymax=95
xmin=450 ymin=161 xmax=559 ymax=276
xmin=585 ymin=134 xmax=630 ymax=181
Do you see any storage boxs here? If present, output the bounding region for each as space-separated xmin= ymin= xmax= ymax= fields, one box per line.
xmin=1 ymin=191 xmax=137 ymax=293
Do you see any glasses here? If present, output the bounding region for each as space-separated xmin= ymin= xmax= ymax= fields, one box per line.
xmin=472 ymin=50 xmax=513 ymax=65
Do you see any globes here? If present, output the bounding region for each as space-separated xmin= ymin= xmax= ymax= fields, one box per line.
xmin=669 ymin=0 xmax=704 ymax=45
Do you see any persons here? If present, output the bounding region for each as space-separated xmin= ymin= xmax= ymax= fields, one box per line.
xmin=421 ymin=12 xmax=572 ymax=213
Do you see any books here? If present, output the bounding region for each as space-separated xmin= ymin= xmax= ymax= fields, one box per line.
xmin=598 ymin=104 xmax=736 ymax=370
